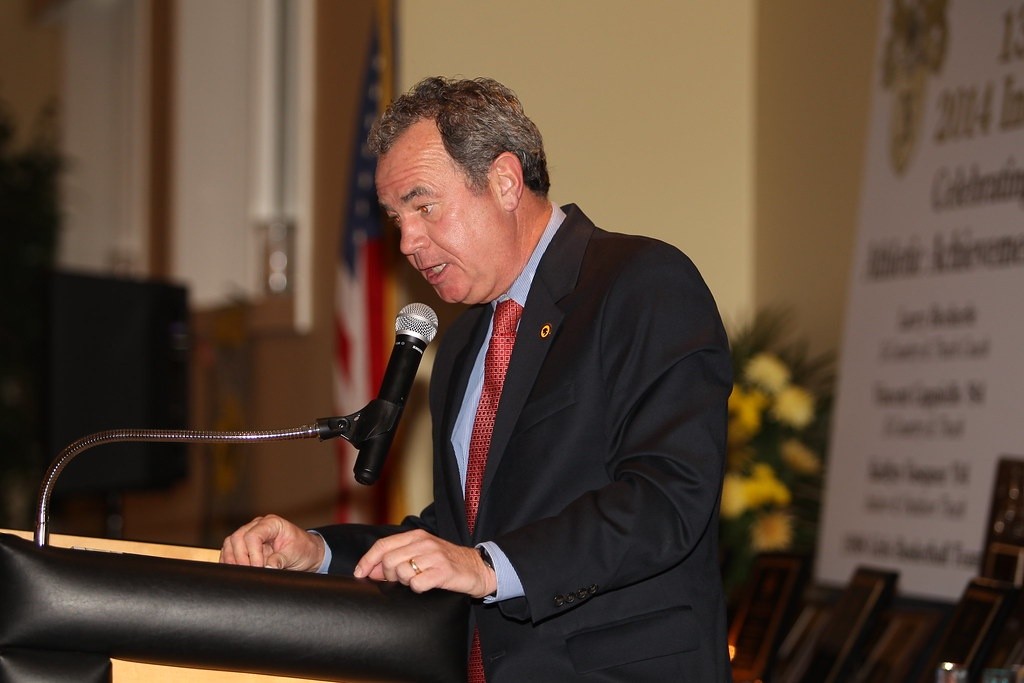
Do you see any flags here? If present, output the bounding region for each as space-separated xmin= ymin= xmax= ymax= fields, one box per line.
xmin=333 ymin=20 xmax=388 ymax=520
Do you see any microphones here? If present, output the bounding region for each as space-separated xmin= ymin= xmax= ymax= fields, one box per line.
xmin=353 ymin=303 xmax=439 ymax=485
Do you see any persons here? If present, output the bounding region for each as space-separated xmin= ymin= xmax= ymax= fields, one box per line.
xmin=218 ymin=77 xmax=732 ymax=683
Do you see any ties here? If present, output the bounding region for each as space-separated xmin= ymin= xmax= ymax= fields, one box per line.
xmin=462 ymin=298 xmax=524 ymax=683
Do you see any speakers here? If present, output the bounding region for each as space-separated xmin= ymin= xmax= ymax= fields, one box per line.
xmin=36 ymin=270 xmax=191 ymax=508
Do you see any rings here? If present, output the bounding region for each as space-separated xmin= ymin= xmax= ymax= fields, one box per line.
xmin=409 ymin=558 xmax=422 ymax=574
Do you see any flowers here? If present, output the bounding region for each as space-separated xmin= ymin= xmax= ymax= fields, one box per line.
xmin=708 ymin=298 xmax=847 ymax=585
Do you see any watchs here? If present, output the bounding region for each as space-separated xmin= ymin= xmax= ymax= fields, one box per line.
xmin=480 ymin=546 xmax=497 ymax=597
